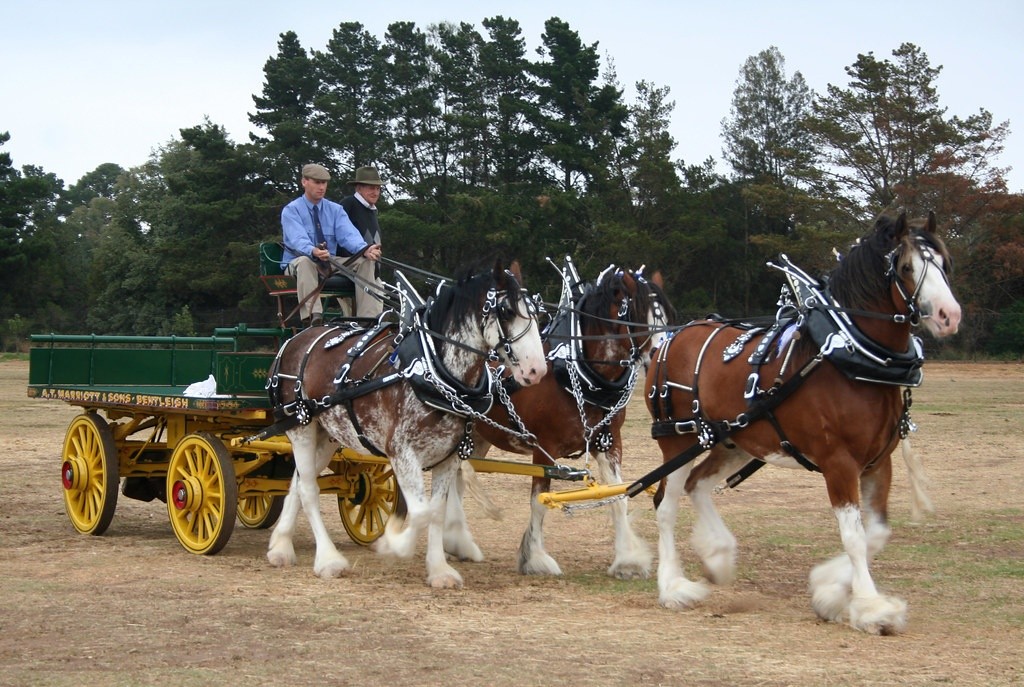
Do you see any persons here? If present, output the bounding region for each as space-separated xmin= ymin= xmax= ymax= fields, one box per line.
xmin=339 ymin=167 xmax=387 ymax=280
xmin=280 ymin=164 xmax=383 ymax=327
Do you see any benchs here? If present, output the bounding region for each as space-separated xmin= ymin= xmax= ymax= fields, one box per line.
xmin=258 ymin=240 xmax=356 ymax=330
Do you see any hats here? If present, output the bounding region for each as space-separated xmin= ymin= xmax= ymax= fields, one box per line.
xmin=346 ymin=167 xmax=387 ymax=186
xmin=303 ymin=164 xmax=331 ymax=180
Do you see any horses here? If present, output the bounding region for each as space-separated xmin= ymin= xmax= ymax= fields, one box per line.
xmin=265 ymin=208 xmax=961 ymax=638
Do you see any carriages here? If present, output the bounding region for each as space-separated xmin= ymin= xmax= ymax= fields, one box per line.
xmin=27 ymin=204 xmax=965 ymax=639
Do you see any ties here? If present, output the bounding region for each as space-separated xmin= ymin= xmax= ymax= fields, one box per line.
xmin=313 ymin=205 xmax=325 ymax=243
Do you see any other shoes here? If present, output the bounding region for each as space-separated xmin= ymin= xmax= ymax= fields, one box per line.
xmin=311 ymin=313 xmax=323 ymax=327
